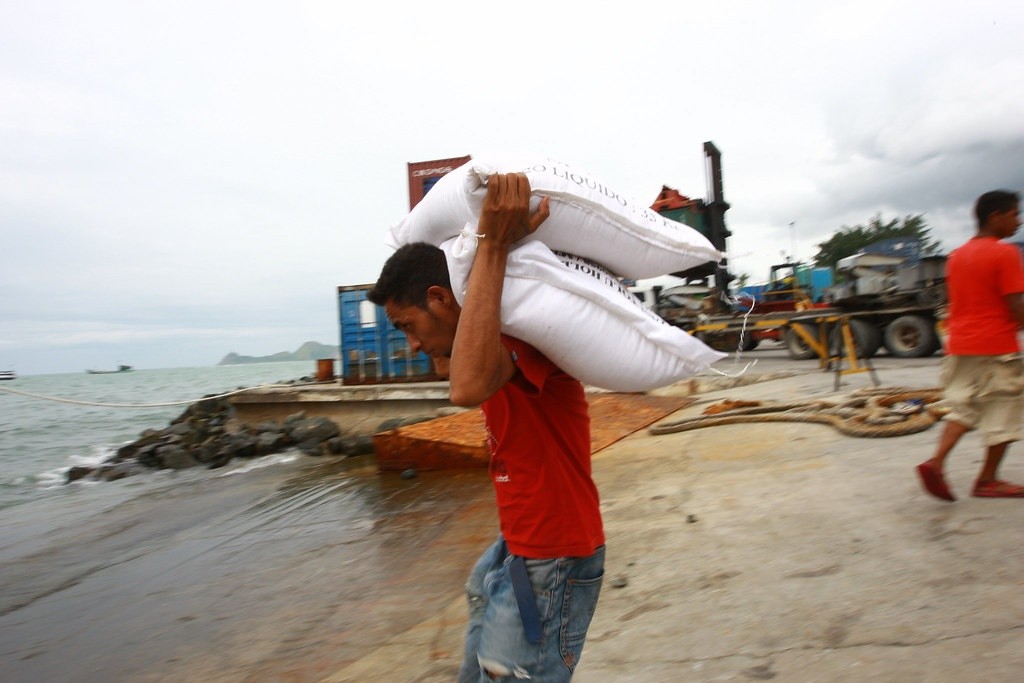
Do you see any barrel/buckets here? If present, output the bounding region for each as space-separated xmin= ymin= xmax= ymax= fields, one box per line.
xmin=318 ymin=359 xmax=333 ymax=381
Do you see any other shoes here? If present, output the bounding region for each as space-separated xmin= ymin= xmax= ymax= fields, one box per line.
xmin=917 ymin=463 xmax=956 ymax=500
xmin=972 ymin=481 xmax=1024 ymax=497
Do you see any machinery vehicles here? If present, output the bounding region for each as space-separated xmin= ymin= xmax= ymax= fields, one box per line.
xmin=732 ymin=263 xmax=835 ymax=350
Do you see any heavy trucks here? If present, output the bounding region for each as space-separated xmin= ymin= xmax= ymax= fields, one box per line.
xmin=627 ymin=251 xmax=1024 ymax=360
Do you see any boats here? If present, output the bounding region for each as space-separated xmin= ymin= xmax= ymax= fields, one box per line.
xmin=1 ymin=371 xmax=17 ymax=381
xmin=88 ymin=366 xmax=132 ymax=373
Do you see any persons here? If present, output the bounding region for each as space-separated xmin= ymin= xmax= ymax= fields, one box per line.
xmin=916 ymin=190 xmax=1024 ymax=500
xmin=366 ymin=172 xmax=606 ymax=683
